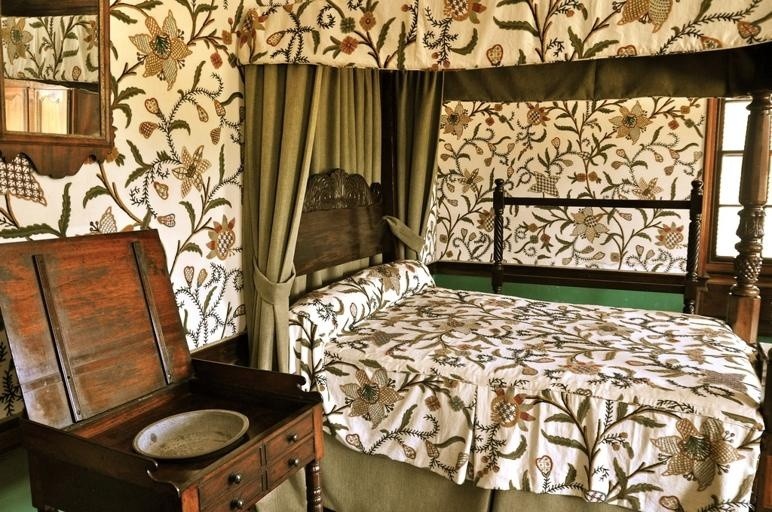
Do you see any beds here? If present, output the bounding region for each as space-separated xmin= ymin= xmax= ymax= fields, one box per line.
xmin=230 ymin=0 xmax=772 ymax=512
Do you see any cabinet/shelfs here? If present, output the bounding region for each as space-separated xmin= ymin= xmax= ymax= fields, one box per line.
xmin=0 ymin=230 xmax=327 ymax=512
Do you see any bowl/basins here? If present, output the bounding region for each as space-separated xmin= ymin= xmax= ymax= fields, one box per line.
xmin=132 ymin=406 xmax=250 ymax=463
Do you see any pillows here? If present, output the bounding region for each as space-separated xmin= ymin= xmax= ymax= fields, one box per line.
xmin=287 ymin=260 xmax=433 ymax=345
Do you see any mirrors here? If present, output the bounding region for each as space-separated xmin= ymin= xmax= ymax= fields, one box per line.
xmin=0 ymin=0 xmax=115 ymax=178
